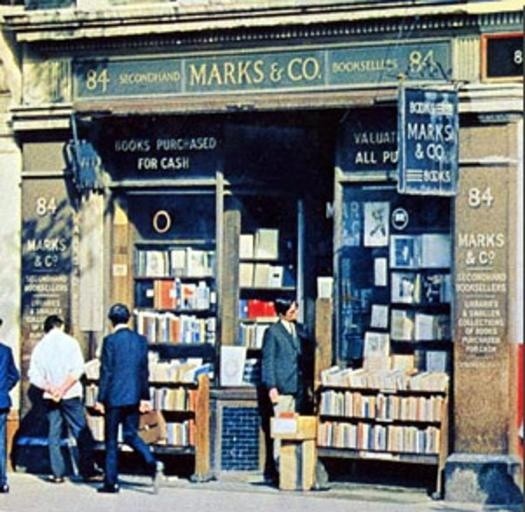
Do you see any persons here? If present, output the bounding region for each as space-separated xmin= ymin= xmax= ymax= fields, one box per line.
xmin=0 ymin=317 xmax=22 ymax=491
xmin=262 ymin=297 xmax=331 ymax=492
xmin=26 ymin=314 xmax=106 ymax=483
xmin=96 ymin=303 xmax=164 ymax=495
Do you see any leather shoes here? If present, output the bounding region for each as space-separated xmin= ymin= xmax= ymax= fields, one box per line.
xmin=151 ymin=460 xmax=165 ymax=494
xmin=98 ymin=482 xmax=119 ymax=493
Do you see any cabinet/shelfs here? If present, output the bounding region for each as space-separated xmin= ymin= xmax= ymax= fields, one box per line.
xmin=129 ymin=201 xmax=240 ymax=350
xmin=76 ymin=358 xmax=216 ymax=482
xmin=315 ymin=365 xmax=452 ymax=496
xmin=383 ymin=225 xmax=455 ymax=366
xmin=240 ymin=199 xmax=305 ymax=354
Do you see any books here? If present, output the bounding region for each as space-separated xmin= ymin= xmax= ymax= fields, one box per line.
xmin=89 ymin=231 xmax=291 ymax=450
xmin=317 ymin=233 xmax=453 ymax=454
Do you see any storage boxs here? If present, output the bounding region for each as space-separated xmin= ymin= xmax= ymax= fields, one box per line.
xmin=269 ymin=413 xmax=319 ymax=441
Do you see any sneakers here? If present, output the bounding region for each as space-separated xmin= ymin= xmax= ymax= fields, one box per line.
xmin=41 ymin=474 xmax=64 ymax=482
xmin=84 ymin=475 xmax=105 ymax=481
